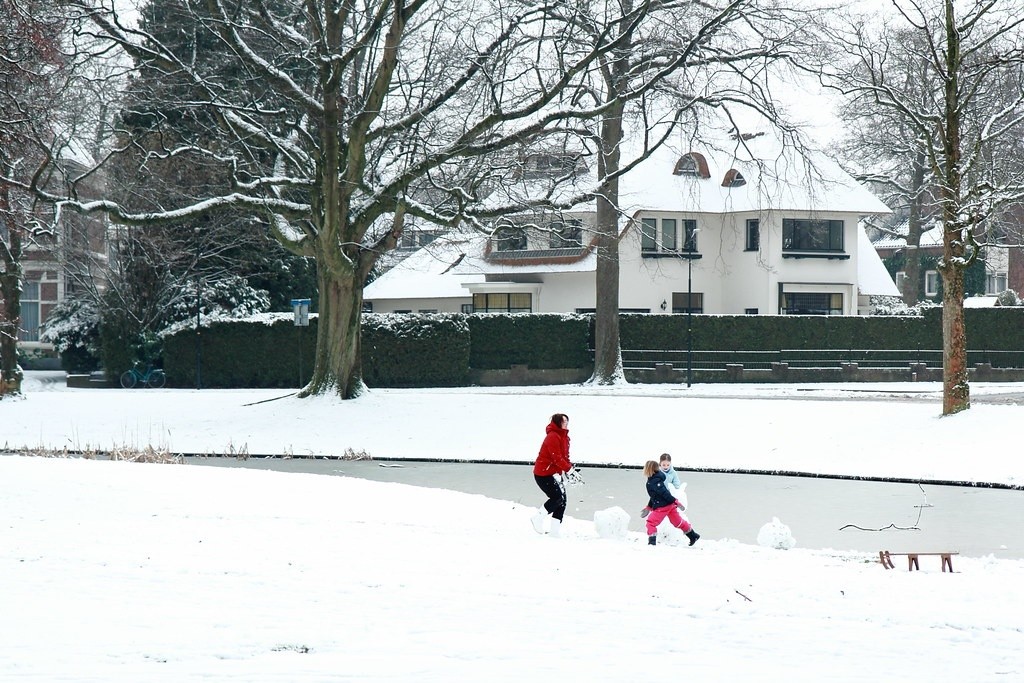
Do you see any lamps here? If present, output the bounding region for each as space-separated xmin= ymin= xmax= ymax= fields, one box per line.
xmin=661 ymin=300 xmax=667 ymax=311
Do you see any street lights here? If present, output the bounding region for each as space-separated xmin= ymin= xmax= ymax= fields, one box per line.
xmin=194 ymin=227 xmax=201 ymax=388
xmin=688 ymin=228 xmax=702 ymax=388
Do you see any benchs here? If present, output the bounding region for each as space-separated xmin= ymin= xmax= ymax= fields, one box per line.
xmin=879 ymin=550 xmax=960 ymax=573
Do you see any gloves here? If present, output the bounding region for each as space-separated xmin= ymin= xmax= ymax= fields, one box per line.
xmin=640 ymin=508 xmax=649 ymax=518
xmin=566 ymin=468 xmax=581 ymax=484
xmin=678 ymin=503 xmax=685 ymax=511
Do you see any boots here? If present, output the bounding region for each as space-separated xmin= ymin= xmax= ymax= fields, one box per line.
xmin=685 ymin=528 xmax=700 ymax=546
xmin=549 ymin=516 xmax=570 ymax=537
xmin=648 ymin=536 xmax=656 ymax=545
xmin=529 ymin=503 xmax=549 ymax=535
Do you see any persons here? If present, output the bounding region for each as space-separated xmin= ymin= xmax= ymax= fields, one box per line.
xmin=641 ymin=452 xmax=701 ymax=546
xmin=529 ymin=414 xmax=582 ymax=536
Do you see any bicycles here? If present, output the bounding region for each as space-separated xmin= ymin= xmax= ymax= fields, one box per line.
xmin=120 ymin=358 xmax=166 ymax=389
